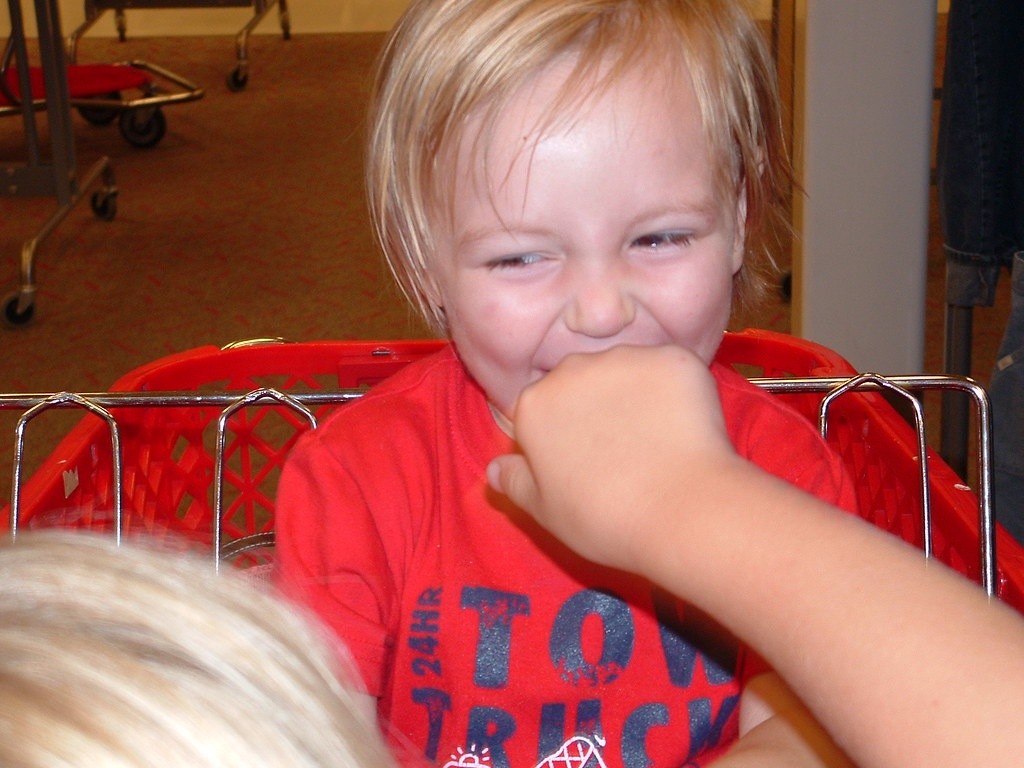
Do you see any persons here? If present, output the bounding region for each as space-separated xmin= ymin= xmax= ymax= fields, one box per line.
xmin=0 ymin=344 xmax=1024 ymax=768
xmin=275 ymin=1 xmax=859 ymax=767
